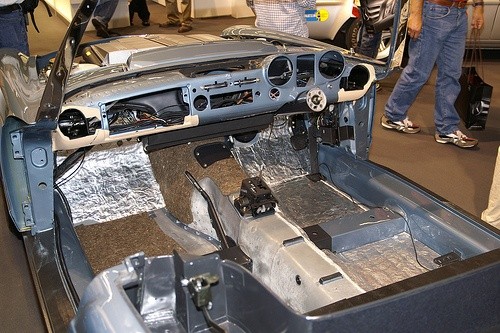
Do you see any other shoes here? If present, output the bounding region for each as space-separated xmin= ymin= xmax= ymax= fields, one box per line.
xmin=142 ymin=21 xmax=150 ymax=26
xmin=130 ymin=22 xmax=134 ymax=25
xmin=91 ymin=16 xmax=111 ymax=38
xmin=374 ymin=83 xmax=382 ymax=92
xmin=178 ymin=24 xmax=192 ymax=33
xmin=159 ymin=19 xmax=181 ymax=28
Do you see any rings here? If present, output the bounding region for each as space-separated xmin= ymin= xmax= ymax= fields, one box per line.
xmin=408 ymin=31 xmax=410 ymax=34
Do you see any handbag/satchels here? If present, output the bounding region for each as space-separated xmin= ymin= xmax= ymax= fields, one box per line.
xmin=454 ymin=26 xmax=493 ymax=130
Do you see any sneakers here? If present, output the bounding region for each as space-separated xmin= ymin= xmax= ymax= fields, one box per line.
xmin=381 ymin=115 xmax=421 ymax=133
xmin=433 ymin=128 xmax=479 ymax=148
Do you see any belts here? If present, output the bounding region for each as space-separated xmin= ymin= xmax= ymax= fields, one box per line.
xmin=3 ymin=5 xmax=21 ymax=14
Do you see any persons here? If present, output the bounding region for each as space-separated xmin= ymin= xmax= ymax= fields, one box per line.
xmin=159 ymin=0 xmax=193 ymax=32
xmin=91 ymin=0 xmax=119 ymax=37
xmin=246 ymin=0 xmax=316 ymax=38
xmin=128 ymin=0 xmax=150 ymax=26
xmin=356 ymin=0 xmax=500 ymax=230
xmin=0 ymin=0 xmax=30 ymax=56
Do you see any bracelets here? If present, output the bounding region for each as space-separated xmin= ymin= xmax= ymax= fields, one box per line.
xmin=472 ymin=1 xmax=483 ymax=7
